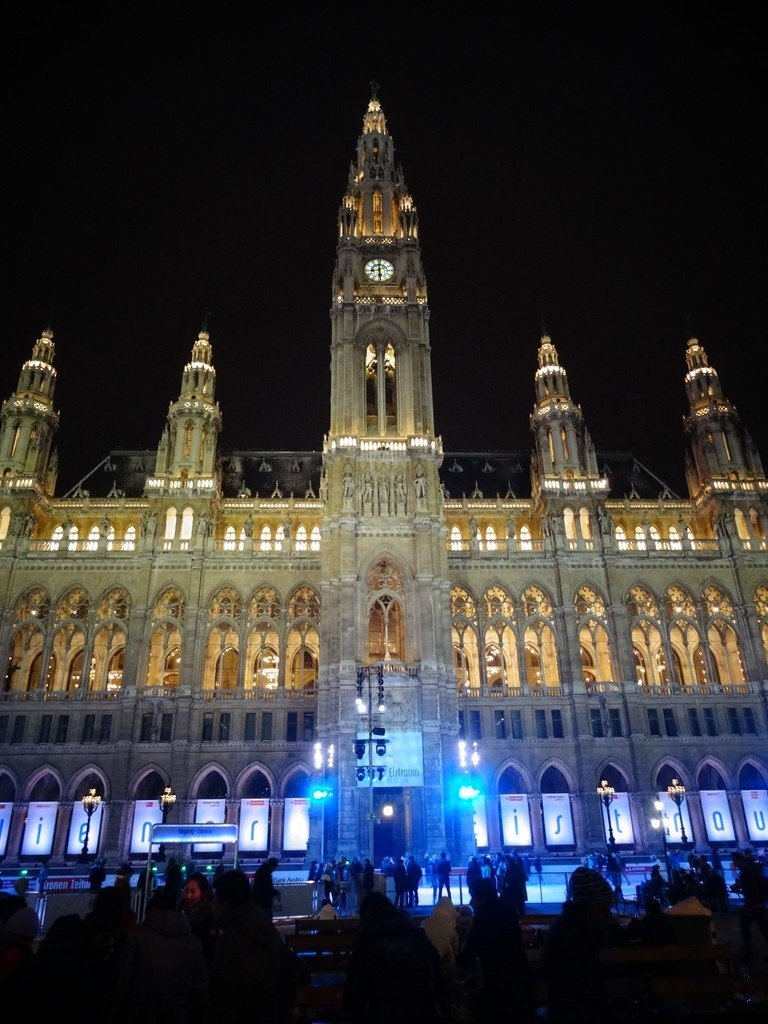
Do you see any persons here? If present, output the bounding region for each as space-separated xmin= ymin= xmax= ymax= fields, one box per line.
xmin=0 ymin=846 xmax=768 ymax=1024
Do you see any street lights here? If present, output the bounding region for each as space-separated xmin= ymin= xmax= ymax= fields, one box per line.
xmin=666 ymin=778 xmax=692 ymax=853
xmin=76 ymin=788 xmax=102 ymax=863
xmin=596 ymin=780 xmax=624 ymax=901
xmin=158 ymin=787 xmax=176 ymax=861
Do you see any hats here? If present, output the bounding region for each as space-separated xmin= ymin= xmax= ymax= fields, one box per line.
xmin=568 ymin=867 xmax=615 ymax=903
